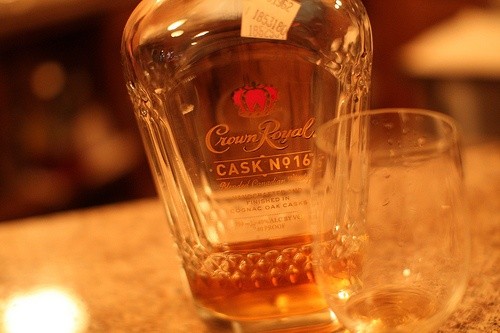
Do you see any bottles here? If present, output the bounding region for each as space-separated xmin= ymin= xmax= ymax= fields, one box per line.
xmin=121 ymin=0 xmax=373 ymax=333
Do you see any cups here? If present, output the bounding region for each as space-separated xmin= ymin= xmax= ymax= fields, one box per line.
xmin=310 ymin=108 xmax=470 ymax=333
